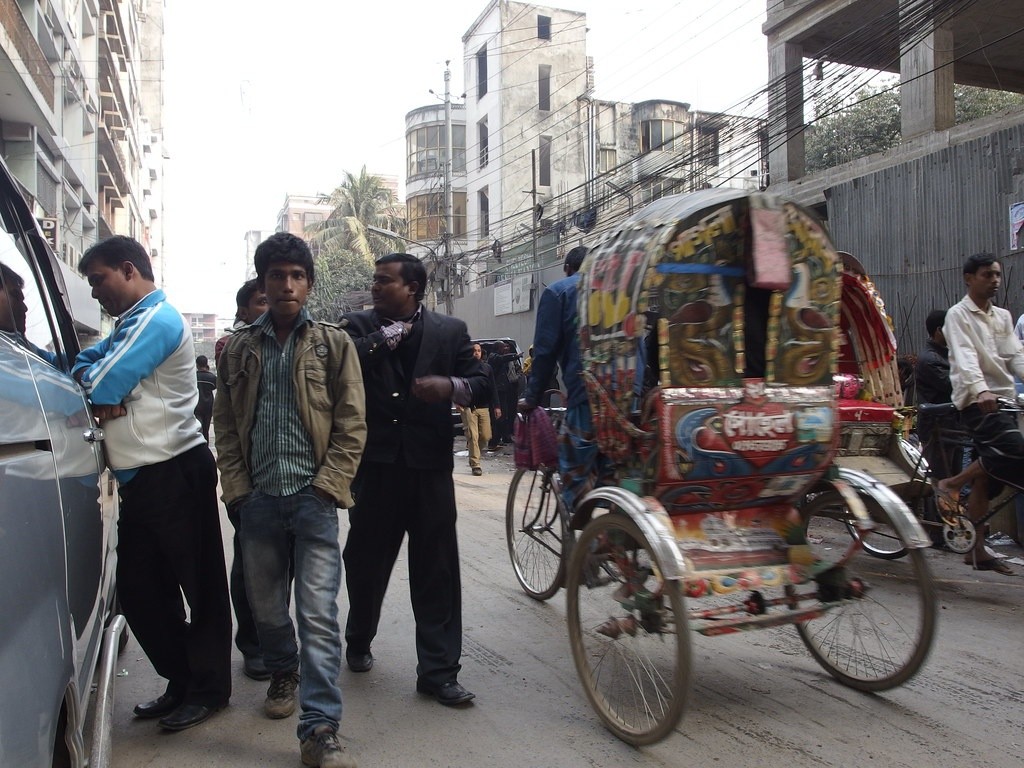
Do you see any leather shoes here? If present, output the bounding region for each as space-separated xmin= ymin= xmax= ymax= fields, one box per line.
xmin=155 ymin=699 xmax=230 ymax=731
xmin=134 ymin=682 xmax=180 ymax=718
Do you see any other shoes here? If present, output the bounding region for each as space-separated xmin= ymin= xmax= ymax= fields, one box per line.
xmin=416 ymin=678 xmax=475 ymax=708
xmin=346 ymin=645 xmax=374 ymax=675
xmin=472 ymin=467 xmax=483 ymax=477
xmin=488 ymin=436 xmax=514 ymax=451
xmin=242 ymin=654 xmax=273 ymax=682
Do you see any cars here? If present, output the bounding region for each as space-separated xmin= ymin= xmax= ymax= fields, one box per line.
xmin=0 ymin=154 xmax=131 ymax=767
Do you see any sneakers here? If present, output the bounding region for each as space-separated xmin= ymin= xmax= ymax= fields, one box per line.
xmin=300 ymin=723 xmax=359 ymax=768
xmin=262 ymin=674 xmax=300 ymax=720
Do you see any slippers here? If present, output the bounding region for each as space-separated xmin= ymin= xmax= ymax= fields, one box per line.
xmin=962 ymin=550 xmax=1015 ymax=577
xmin=931 ymin=477 xmax=962 ymax=525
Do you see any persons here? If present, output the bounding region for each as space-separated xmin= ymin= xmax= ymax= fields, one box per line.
xmin=489 ymin=341 xmax=524 ymax=451
xmin=71 ymin=235 xmax=233 ymax=731
xmin=0 ymin=262 xmax=105 ymax=640
xmin=897 ymin=357 xmax=912 ymax=384
xmin=214 ymin=234 xmax=367 ymax=768
xmin=454 ymin=343 xmax=501 ymax=476
xmin=933 ymin=252 xmax=1024 ymax=576
xmin=517 ymin=246 xmax=647 ymax=514
xmin=1012 ymin=314 xmax=1024 ymax=550
xmin=915 ymin=308 xmax=965 ymax=552
xmin=215 ymin=279 xmax=294 ymax=680
xmin=339 ymin=253 xmax=490 ymax=706
xmin=523 ymin=345 xmax=533 ymax=384
xmin=194 ymin=355 xmax=217 ymax=446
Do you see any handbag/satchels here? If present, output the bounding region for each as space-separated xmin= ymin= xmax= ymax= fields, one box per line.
xmin=513 ymin=405 xmax=558 ymax=471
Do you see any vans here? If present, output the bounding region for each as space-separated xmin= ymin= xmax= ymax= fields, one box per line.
xmin=449 ymin=337 xmax=530 ymax=437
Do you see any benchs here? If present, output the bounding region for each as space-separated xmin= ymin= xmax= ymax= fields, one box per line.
xmin=837 ymin=398 xmax=895 ymax=426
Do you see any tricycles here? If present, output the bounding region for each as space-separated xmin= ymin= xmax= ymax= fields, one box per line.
xmin=505 ymin=188 xmax=939 ymax=746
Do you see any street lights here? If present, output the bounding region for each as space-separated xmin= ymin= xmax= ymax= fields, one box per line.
xmin=367 ymin=224 xmax=454 ymax=316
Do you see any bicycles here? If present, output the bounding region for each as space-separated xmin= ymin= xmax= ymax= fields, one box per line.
xmin=842 ymin=396 xmax=1024 ymax=573
